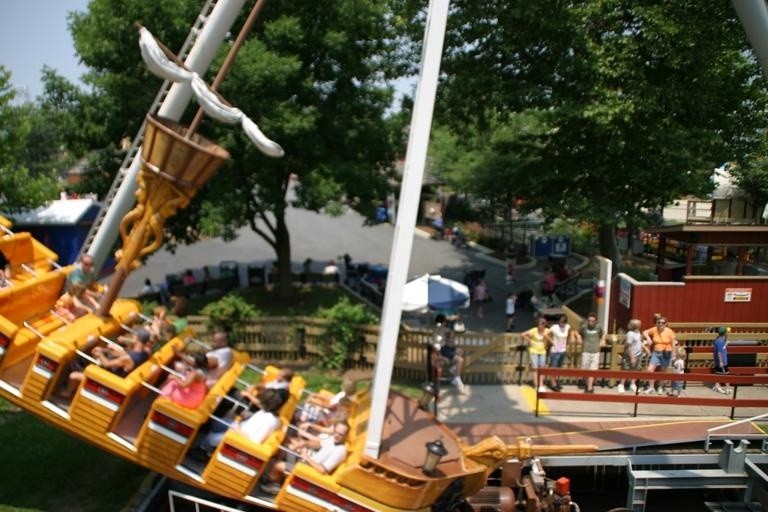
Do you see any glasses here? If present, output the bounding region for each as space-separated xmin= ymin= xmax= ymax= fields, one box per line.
xmin=658 ymin=322 xmax=666 ymax=324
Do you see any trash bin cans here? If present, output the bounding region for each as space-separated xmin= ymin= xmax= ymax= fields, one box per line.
xmin=550 ymin=255 xmax=567 ymax=272
xmin=247 ymin=264 xmax=266 ymax=289
xmin=221 ymin=261 xmax=240 ymax=288
xmin=726 ymin=340 xmax=760 ymax=386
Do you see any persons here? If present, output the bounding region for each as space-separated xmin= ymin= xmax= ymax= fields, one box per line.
xmin=544 ymin=262 xmax=569 ymax=293
xmin=449 ymin=231 xmax=466 ymax=251
xmin=60 ymin=187 xmax=67 ymax=200
xmin=506 ymin=258 xmax=515 ymax=285
xmin=522 ymin=312 xmax=605 ymax=393
xmin=505 ymin=291 xmax=519 ymax=332
xmin=432 ymin=314 xmax=465 ymax=390
xmin=71 ymin=191 xmax=77 ymax=199
xmin=713 ymin=326 xmax=732 ymax=395
xmin=618 ymin=317 xmax=687 ymax=397
xmin=517 ymin=280 xmax=534 ymax=315
xmin=474 ymin=278 xmax=489 ymax=319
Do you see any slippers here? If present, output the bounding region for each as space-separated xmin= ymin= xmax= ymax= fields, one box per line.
xmin=548 ymin=383 xmax=562 ymax=392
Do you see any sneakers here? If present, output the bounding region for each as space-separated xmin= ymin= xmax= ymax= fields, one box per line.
xmin=584 ymin=385 xmax=593 ymax=394
xmin=449 ymin=365 xmax=457 ymax=375
xmin=450 ymin=377 xmax=464 ymax=391
xmin=616 ymin=383 xmax=683 ymax=398
xmin=713 ymin=386 xmax=733 ymax=395
xmin=535 ymin=386 xmax=547 ymax=392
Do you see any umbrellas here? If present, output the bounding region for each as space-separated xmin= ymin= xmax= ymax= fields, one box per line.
xmin=402 ymin=273 xmax=471 ymax=343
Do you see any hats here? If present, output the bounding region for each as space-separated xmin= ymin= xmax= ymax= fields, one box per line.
xmin=717 ymin=326 xmax=732 ymax=335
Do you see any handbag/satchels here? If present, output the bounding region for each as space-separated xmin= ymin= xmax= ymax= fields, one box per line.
xmin=663 ymin=350 xmax=672 ymax=359
